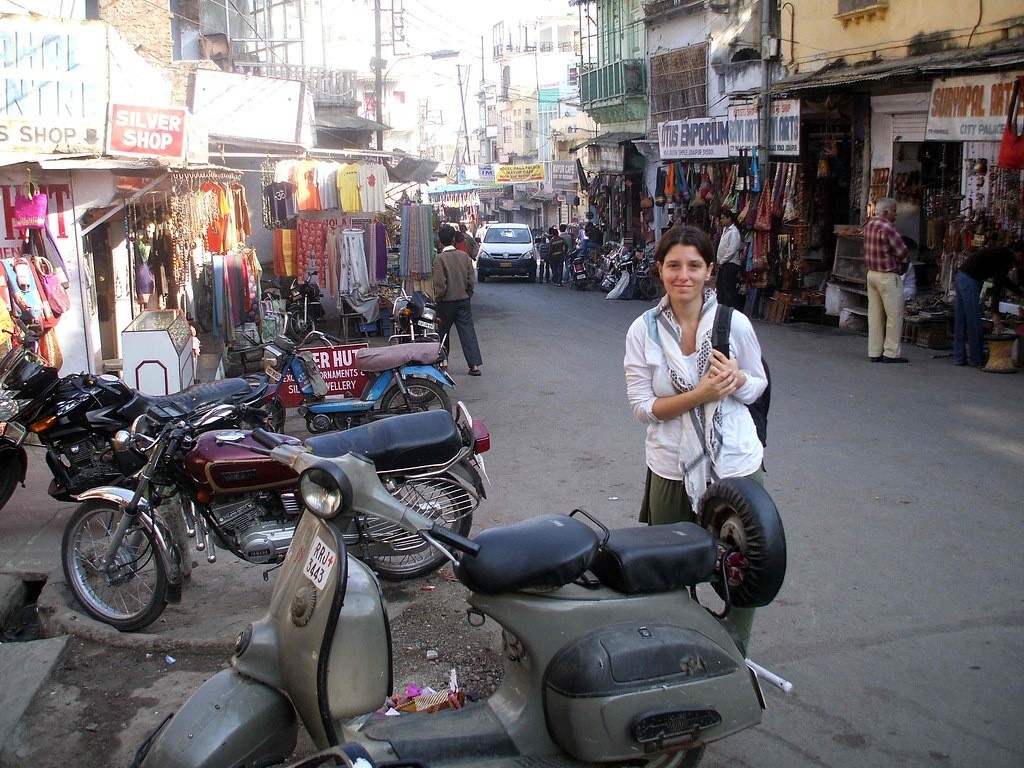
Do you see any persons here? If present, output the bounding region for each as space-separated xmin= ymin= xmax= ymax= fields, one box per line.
xmin=863 ymin=198 xmax=911 ymax=363
xmin=952 ymin=243 xmax=1024 ymax=368
xmin=621 ymin=228 xmax=768 ymax=652
xmin=431 ymin=225 xmax=484 ymax=376
xmin=433 ymin=223 xmax=478 ymax=260
xmin=536 ymin=221 xmax=603 ymax=286
xmin=715 ymin=208 xmax=740 ymax=306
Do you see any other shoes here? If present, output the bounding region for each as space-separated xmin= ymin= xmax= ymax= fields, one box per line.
xmin=468 ymin=368 xmax=481 ymax=376
xmin=540 ymin=281 xmax=544 ymax=283
xmin=546 ymin=280 xmax=551 ymax=283
xmin=553 ymin=283 xmax=563 ymax=287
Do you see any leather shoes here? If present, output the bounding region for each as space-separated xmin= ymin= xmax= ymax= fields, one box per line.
xmin=881 ymin=354 xmax=909 ymax=364
xmin=870 ymin=355 xmax=882 ymax=363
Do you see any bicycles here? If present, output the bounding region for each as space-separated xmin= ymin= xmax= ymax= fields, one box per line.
xmin=197 ymin=262 xmax=217 ymax=334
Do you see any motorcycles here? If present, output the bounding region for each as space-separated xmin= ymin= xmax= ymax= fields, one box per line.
xmin=260 ymin=268 xmax=327 ymax=344
xmin=375 ymin=281 xmax=449 ymax=404
xmin=0 ymin=317 xmax=275 ymax=510
xmin=251 ymin=329 xmax=459 ymax=435
xmin=60 ymin=402 xmax=492 ymax=634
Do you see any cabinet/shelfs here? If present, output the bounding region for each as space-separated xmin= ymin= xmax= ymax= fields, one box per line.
xmin=832 ymin=236 xmax=866 ymax=284
xmin=121 ymin=310 xmax=194 ymax=396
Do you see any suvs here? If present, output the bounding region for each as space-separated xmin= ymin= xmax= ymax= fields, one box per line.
xmin=474 ymin=222 xmax=542 ymax=283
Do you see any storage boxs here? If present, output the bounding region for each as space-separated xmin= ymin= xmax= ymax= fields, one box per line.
xmin=357 ymin=307 xmax=391 ymax=336
xmin=542 ymin=619 xmax=762 ymax=762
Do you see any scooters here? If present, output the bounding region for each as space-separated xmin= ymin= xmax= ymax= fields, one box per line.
xmin=128 ymin=427 xmax=797 ymax=768
xmin=562 ymin=235 xmax=661 ymax=301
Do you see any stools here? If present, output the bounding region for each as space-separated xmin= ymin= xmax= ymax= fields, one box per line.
xmin=338 ymin=313 xmax=368 ymax=345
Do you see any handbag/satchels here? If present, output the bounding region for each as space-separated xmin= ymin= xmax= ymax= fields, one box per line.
xmin=13 ymin=180 xmax=49 ymax=230
xmin=33 ymin=255 xmax=72 ymax=317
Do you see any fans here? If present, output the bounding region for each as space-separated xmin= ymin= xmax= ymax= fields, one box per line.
xmin=94 ymin=253 xmax=112 ymax=295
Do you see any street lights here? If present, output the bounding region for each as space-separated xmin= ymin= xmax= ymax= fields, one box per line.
xmin=372 ymin=46 xmax=461 ymax=151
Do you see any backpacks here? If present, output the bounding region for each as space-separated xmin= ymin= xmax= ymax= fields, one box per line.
xmin=711 ymin=302 xmax=772 ymax=472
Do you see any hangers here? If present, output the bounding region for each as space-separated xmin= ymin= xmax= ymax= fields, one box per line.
xmin=176 ymin=172 xmax=242 ymax=195
xmin=264 ymin=148 xmax=376 ymax=165
